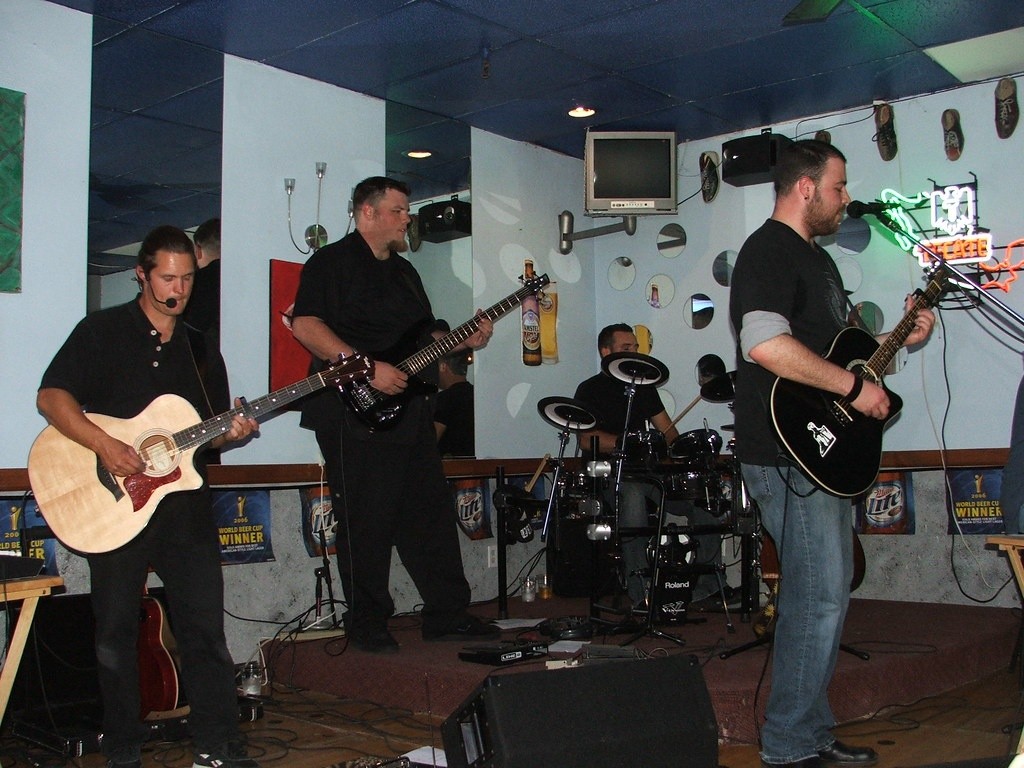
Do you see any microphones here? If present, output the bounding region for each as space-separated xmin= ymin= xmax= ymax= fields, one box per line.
xmin=144 ymin=272 xmax=177 ymax=308
xmin=847 ymin=200 xmax=901 ymax=219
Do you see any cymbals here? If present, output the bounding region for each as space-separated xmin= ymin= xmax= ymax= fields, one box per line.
xmin=700 ymin=370 xmax=740 ymax=403
xmin=599 ymin=352 xmax=674 ymax=388
xmin=537 ymin=396 xmax=606 ymax=434
xmin=720 ymin=425 xmax=736 ymax=431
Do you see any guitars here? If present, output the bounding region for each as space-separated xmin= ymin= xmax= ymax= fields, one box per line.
xmin=28 ymin=350 xmax=376 ymax=557
xmin=768 ymin=260 xmax=958 ymax=500
xmin=337 ymin=271 xmax=552 ymax=439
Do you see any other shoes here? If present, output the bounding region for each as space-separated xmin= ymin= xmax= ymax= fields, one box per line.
xmin=420 ymin=612 xmax=502 ymax=640
xmin=713 ymin=587 xmax=744 ymax=608
xmin=631 ymin=601 xmax=650 ymax=615
xmin=343 ymin=619 xmax=398 ymax=654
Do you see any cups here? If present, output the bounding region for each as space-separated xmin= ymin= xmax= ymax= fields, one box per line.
xmin=521 ymin=576 xmax=535 ymax=603
xmin=539 ymin=282 xmax=559 ymax=365
xmin=537 ymin=573 xmax=553 ymax=600
xmin=239 ymin=664 xmax=264 ymax=696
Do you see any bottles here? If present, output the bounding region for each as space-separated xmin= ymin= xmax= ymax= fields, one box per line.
xmin=520 ymin=260 xmax=542 ymax=366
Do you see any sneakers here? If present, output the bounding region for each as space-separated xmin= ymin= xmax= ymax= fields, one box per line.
xmin=191 ymin=750 xmax=264 ymax=768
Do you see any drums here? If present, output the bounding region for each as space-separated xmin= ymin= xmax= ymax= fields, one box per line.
xmin=669 ymin=428 xmax=723 ymax=460
xmin=558 ymin=448 xmax=743 ymax=605
xmin=615 ymin=428 xmax=669 ymax=473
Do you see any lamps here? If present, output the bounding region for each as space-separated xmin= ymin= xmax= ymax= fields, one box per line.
xmin=285 ymin=162 xmax=354 ymax=254
xmin=567 ymin=96 xmax=595 ymax=118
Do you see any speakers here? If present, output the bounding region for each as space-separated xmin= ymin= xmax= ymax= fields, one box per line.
xmin=418 ymin=200 xmax=472 ymax=243
xmin=440 ymin=654 xmax=720 ymax=768
xmin=722 ymin=133 xmax=796 ymax=187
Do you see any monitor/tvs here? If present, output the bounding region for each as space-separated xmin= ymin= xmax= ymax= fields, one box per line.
xmin=586 ymin=131 xmax=678 ymax=215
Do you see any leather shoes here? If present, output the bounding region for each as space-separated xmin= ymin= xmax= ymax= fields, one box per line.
xmin=819 ymin=738 xmax=880 ymax=766
xmin=760 ymin=751 xmax=828 ymax=768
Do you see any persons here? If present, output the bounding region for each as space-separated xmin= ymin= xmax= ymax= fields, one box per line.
xmin=37 ymin=227 xmax=261 ymax=768
xmin=999 ymin=375 xmax=1023 ymax=530
xmin=431 ymin=319 xmax=450 ymax=340
xmin=434 ymin=351 xmax=475 ymax=457
xmin=183 ymin=218 xmax=221 ymax=350
xmin=731 ymin=138 xmax=936 ymax=768
xmin=574 ymin=323 xmax=743 ymax=613
xmin=290 ymin=176 xmax=502 ymax=650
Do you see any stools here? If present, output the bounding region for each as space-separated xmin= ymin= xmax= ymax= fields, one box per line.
xmin=985 ymin=536 xmax=1024 ymax=599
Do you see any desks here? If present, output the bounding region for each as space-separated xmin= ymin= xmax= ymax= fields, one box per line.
xmin=0 ymin=576 xmax=64 ymax=729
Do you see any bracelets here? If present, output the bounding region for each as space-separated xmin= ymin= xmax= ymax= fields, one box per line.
xmin=844 ymin=375 xmax=864 ymax=402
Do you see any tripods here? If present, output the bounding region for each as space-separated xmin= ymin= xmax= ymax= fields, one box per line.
xmin=614 ymin=472 xmax=688 ymax=647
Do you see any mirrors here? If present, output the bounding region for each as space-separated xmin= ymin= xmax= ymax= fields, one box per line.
xmin=607 ymin=257 xmax=636 ymax=291
xmin=682 ymin=293 xmax=714 ymax=329
xmin=694 ymin=354 xmax=726 ymax=387
xmin=657 ymin=223 xmax=687 ymax=258
xmin=657 ymin=388 xmax=676 ymax=420
xmin=645 ymin=274 xmax=675 ymax=308
xmin=87 ymin=15 xmax=224 ymax=356
xmin=385 ymin=99 xmax=475 ymax=456
xmin=835 ymin=216 xmax=871 ymax=255
xmin=834 ymin=257 xmax=862 ymax=295
xmin=855 ymin=301 xmax=884 ymax=337
xmin=712 ymin=250 xmax=739 ymax=287
xmin=630 ymin=325 xmax=654 ymax=355
